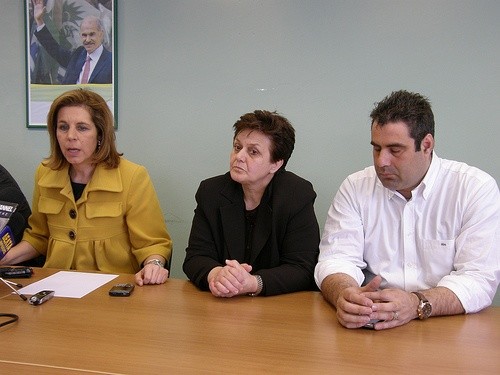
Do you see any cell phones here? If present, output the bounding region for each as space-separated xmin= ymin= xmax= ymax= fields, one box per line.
xmin=109 ymin=283 xmax=134 ymax=296
xmin=0 ymin=267 xmax=33 ymax=278
xmin=29 ymin=291 xmax=54 ymax=305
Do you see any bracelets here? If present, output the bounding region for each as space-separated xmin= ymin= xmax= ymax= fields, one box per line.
xmin=143 ymin=259 xmax=167 ymax=269
xmin=247 ymin=275 xmax=263 ymax=296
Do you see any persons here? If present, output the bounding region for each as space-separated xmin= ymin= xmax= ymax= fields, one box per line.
xmin=0 ymin=90 xmax=174 ymax=287
xmin=313 ymin=88 xmax=500 ymax=330
xmin=181 ymin=109 xmax=321 ymax=299
xmin=28 ymin=0 xmax=112 ymax=84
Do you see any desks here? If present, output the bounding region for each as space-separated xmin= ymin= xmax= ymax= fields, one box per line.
xmin=0 ymin=266 xmax=500 ymax=375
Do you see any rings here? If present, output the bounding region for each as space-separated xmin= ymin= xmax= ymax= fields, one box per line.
xmin=391 ymin=311 xmax=399 ymax=320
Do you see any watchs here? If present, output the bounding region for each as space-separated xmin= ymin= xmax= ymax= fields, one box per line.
xmin=411 ymin=291 xmax=432 ymax=320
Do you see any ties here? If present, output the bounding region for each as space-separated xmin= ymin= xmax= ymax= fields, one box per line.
xmin=81 ymin=56 xmax=91 ymax=84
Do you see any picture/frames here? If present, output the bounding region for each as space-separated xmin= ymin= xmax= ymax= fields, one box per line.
xmin=24 ymin=0 xmax=120 ymax=129
